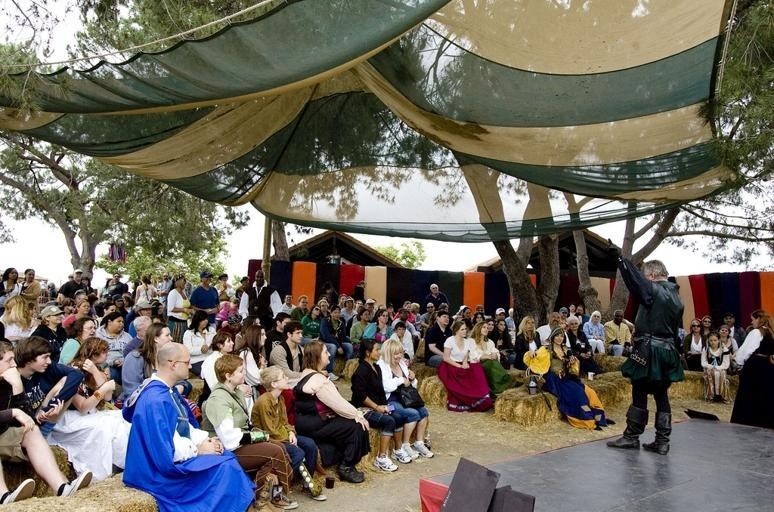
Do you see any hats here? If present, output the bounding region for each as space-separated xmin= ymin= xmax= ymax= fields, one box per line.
xmin=200 ymin=270 xmax=213 ymax=278
xmin=560 ymin=307 xmax=568 ymax=314
xmin=112 ymin=294 xmax=124 ymax=301
xmin=496 ymin=308 xmax=506 ymax=314
xmin=135 ymin=301 xmax=156 ymax=314
xmin=366 ymin=298 xmax=375 ymax=304
xmin=41 ymin=305 xmax=64 ymax=320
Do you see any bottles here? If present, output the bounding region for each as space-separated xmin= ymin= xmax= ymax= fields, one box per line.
xmin=527 ymin=378 xmax=538 ymax=395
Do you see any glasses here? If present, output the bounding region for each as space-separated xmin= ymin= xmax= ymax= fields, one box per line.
xmin=168 ymin=359 xmax=190 ymax=366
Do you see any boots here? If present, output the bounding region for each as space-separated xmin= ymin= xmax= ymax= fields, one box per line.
xmin=606 ymin=404 xmax=648 ymax=449
xmin=643 ymin=412 xmax=672 ymax=455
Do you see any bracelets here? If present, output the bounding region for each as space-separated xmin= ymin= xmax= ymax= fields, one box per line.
xmin=91 ymin=389 xmax=104 ymax=404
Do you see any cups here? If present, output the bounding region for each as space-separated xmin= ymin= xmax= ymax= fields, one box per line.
xmin=326 ymin=477 xmax=335 ymax=490
xmin=587 ymin=372 xmax=595 ymax=381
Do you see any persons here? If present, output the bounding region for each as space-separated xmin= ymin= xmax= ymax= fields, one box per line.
xmin=419 ymin=284 xmax=634 ymax=431
xmin=601 ymin=234 xmax=684 ymax=459
xmin=0 ymin=268 xmax=434 ymax=512
xmin=57 ymin=338 xmax=131 ymax=486
xmin=123 ymin=342 xmax=261 ymax=512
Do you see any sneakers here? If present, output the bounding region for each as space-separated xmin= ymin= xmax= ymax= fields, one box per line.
xmin=60 ymin=471 xmax=92 ymax=497
xmin=271 ymin=442 xmax=434 ymax=510
xmin=328 ymin=372 xmax=339 ymax=381
xmin=2 ymin=479 xmax=35 ymax=504
